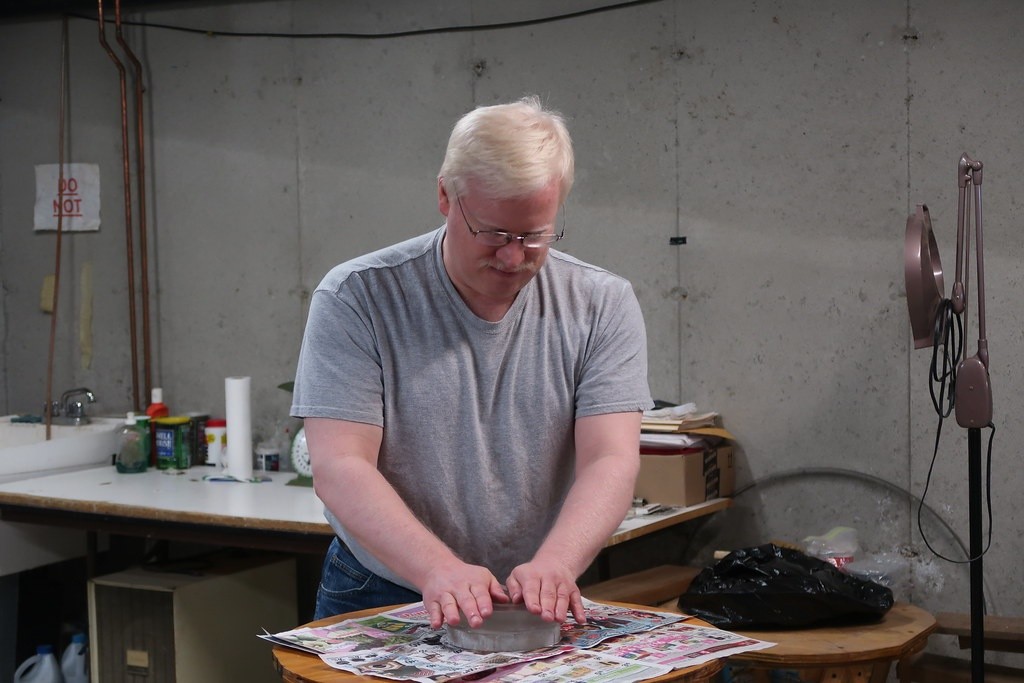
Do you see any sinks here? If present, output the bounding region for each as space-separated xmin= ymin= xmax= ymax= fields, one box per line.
xmin=0 ymin=421 xmax=70 ymax=452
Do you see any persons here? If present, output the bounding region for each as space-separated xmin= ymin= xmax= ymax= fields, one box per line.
xmin=290 ymin=99 xmax=653 ymax=627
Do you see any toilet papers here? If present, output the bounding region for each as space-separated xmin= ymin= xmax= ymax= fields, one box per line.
xmin=223 ymin=374 xmax=252 ymax=477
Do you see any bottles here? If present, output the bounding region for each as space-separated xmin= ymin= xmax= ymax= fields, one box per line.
xmin=62 ymin=634 xmax=90 ymax=683
xmin=147 ymin=389 xmax=169 ymax=465
xmin=13 ymin=646 xmax=62 ymax=683
xmin=117 ymin=413 xmax=147 ymax=474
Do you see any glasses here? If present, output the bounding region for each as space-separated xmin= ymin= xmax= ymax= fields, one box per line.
xmin=452 ymin=179 xmax=566 ymax=248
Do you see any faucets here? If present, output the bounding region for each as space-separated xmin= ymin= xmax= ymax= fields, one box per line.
xmin=39 ymin=387 xmax=95 ymax=418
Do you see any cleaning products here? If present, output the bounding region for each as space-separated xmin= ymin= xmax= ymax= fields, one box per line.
xmin=115 ymin=410 xmax=151 ymax=474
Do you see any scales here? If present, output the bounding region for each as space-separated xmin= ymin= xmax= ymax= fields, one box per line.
xmin=277 ymin=381 xmax=314 ymax=488
